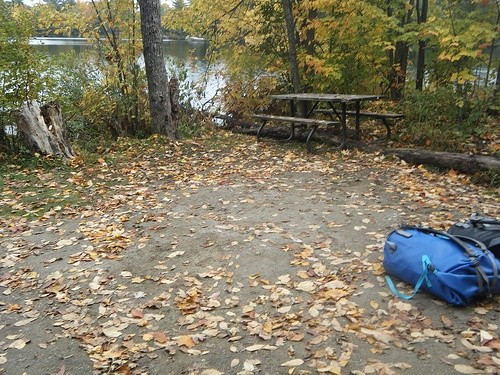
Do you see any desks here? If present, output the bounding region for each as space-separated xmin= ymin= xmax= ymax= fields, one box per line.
xmin=269 ymin=92 xmax=382 ymax=138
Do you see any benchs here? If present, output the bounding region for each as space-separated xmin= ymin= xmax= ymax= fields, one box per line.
xmin=310 ymin=107 xmax=403 ymax=139
xmin=248 ymin=110 xmax=344 ymax=153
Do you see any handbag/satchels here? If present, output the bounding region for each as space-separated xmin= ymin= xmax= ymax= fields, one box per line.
xmin=447 ymin=216 xmax=500 ymax=260
xmin=383 ymin=226 xmax=500 ymax=307
